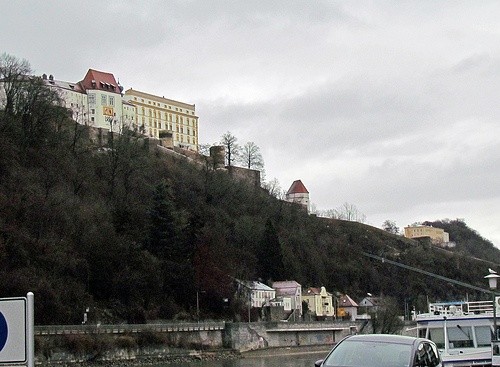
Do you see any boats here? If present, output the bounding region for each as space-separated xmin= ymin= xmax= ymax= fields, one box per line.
xmin=405 ymin=292 xmax=500 ymax=366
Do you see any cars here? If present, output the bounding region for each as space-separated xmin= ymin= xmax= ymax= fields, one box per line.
xmin=314 ymin=332 xmax=444 ymax=366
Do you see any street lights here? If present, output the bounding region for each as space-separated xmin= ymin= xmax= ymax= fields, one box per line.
xmin=483 ymin=272 xmax=500 ymax=342
